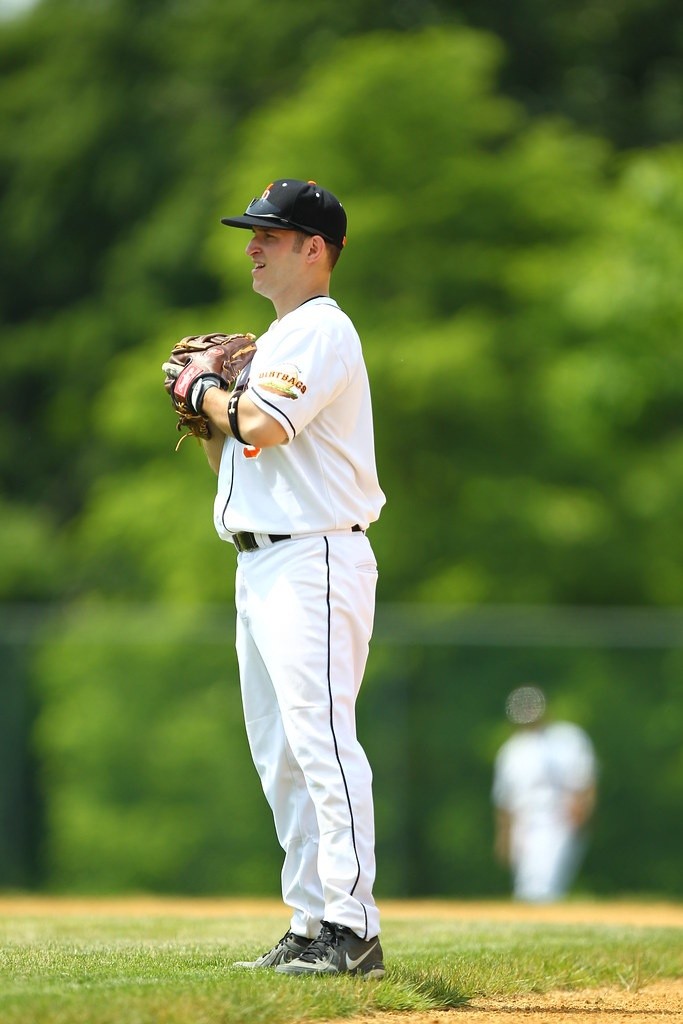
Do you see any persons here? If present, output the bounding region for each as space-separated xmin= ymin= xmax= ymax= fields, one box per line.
xmin=492 ymin=684 xmax=597 ymax=901
xmin=162 ymin=179 xmax=386 ymax=983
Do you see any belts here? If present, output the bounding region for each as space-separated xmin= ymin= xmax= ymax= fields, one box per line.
xmin=232 ymin=524 xmax=362 ymax=552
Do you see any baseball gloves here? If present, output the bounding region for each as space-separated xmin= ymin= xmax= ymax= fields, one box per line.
xmin=163 ymin=329 xmax=258 ymax=440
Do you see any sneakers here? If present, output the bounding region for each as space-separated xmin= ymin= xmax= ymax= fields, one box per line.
xmin=233 ymin=919 xmax=386 ymax=981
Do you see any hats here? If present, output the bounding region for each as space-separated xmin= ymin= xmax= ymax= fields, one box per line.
xmin=221 ymin=179 xmax=347 ymax=249
